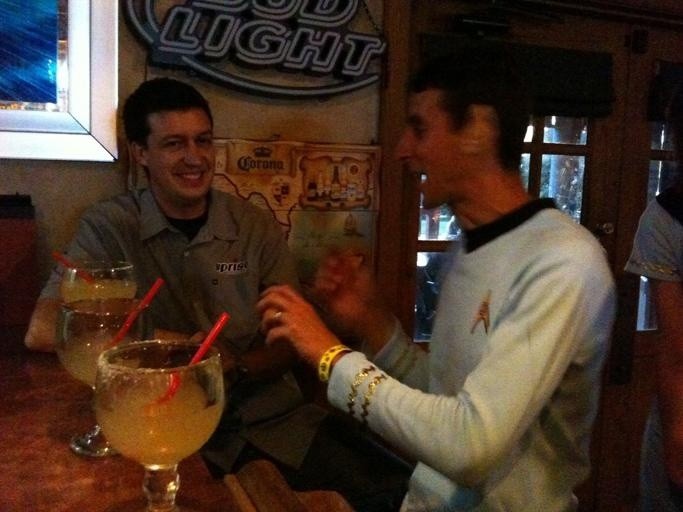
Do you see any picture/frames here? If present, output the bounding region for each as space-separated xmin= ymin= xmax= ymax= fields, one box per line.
xmin=0 ymin=0 xmax=119 ymax=162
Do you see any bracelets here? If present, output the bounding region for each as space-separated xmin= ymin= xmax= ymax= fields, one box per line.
xmin=318 ymin=343 xmax=352 ymax=383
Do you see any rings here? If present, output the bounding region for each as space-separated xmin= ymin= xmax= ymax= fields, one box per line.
xmin=274 ymin=310 xmax=282 ymax=322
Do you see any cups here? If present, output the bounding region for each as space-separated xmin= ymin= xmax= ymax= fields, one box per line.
xmin=53 ymin=296 xmax=153 ymax=461
xmin=59 ymin=259 xmax=139 ymax=305
xmin=92 ymin=337 xmax=227 ymax=512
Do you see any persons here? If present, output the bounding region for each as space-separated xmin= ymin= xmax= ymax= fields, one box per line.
xmin=256 ymin=63 xmax=617 ymax=511
xmin=623 ymin=68 xmax=682 ymax=512
xmin=23 ymin=78 xmax=413 ymax=512
